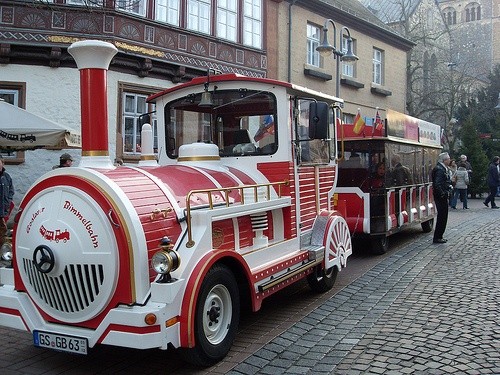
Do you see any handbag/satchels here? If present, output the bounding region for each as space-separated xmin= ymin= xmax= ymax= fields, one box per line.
xmin=451 ymin=171 xmax=457 ymax=186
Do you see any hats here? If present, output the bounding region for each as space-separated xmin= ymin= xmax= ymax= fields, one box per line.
xmin=59 ymin=153 xmax=76 ymax=161
xmin=439 ymin=152 xmax=450 ymax=160
xmin=391 ymin=153 xmax=401 ymax=162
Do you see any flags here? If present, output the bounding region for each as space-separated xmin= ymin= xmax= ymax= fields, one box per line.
xmin=352 ymin=114 xmax=365 ymax=137
xmin=374 ymin=113 xmax=382 ymax=137
xmin=253 ymin=115 xmax=274 ymax=143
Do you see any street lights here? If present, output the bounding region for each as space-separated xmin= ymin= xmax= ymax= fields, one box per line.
xmin=315 ymin=18 xmax=358 ymax=119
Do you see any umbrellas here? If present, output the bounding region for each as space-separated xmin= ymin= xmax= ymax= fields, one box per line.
xmin=0 ymin=98 xmax=80 ymax=151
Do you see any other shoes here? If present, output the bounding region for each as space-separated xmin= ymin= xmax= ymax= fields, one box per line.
xmin=483 ymin=202 xmax=489 ymax=207
xmin=491 ymin=206 xmax=500 ymax=209
xmin=434 ymin=238 xmax=447 ymax=243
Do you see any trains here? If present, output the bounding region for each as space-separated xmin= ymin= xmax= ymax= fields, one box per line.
xmin=0 ymin=40 xmax=443 ymax=358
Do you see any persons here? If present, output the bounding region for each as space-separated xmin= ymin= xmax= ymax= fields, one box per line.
xmin=483 ymin=156 xmax=500 ymax=209
xmin=0 ymin=154 xmax=15 ymax=254
xmin=52 ymin=153 xmax=76 ymax=169
xmin=449 ymin=155 xmax=472 ymax=209
xmin=432 ymin=152 xmax=455 ymax=244
